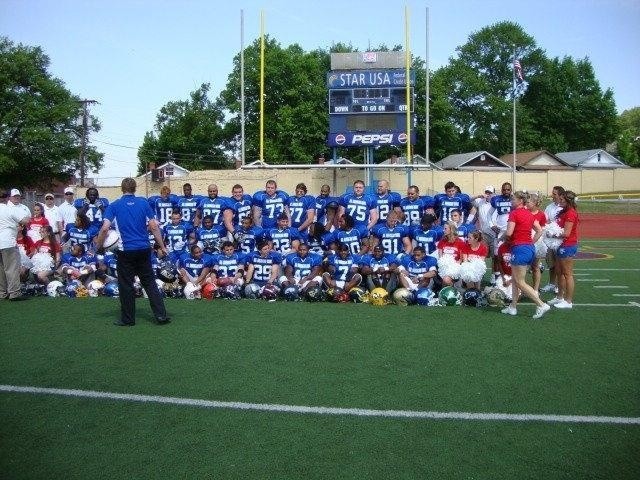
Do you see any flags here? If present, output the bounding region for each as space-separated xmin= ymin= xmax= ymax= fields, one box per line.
xmin=514 ymin=49 xmax=523 ymax=83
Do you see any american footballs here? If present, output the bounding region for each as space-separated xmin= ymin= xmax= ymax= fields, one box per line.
xmin=217 ymin=277 xmax=228 ymax=285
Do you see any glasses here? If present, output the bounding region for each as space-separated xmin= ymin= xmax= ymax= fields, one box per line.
xmin=65 ymin=193 xmax=73 ymax=195
xmin=46 ymin=198 xmax=54 ymax=200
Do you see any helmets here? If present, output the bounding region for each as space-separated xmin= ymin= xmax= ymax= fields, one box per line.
xmin=414 ymin=288 xmax=436 ymax=308
xmin=46 ymin=280 xmax=64 ymax=298
xmin=462 ymin=287 xmax=484 ymax=308
xmin=86 ymin=279 xmax=105 ymax=298
xmin=104 ymin=281 xmax=119 ymax=298
xmin=392 ymin=287 xmax=415 ymax=308
xmin=529 ymin=261 xmax=546 ymax=274
xmin=485 ymin=288 xmax=507 ymax=310
xmin=438 ymin=285 xmax=464 ymax=308
xmin=184 ymin=281 xmax=389 ymax=307
xmin=132 ymin=282 xmax=144 ymax=298
xmin=65 ymin=282 xmax=79 ymax=298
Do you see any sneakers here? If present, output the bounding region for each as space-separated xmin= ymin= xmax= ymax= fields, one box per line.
xmin=539 ymin=283 xmax=559 ymax=293
xmin=10 ymin=295 xmax=29 ymax=301
xmin=113 ymin=319 xmax=135 ymax=326
xmin=490 ymin=274 xmax=497 ymax=284
xmin=553 ymin=300 xmax=573 ymax=309
xmin=501 ymin=305 xmax=518 ymax=316
xmin=547 ymin=296 xmax=565 ymax=306
xmin=532 ymin=302 xmax=551 ymax=319
xmin=157 ymin=317 xmax=172 ymax=325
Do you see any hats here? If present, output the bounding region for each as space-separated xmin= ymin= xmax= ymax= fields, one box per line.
xmin=10 ymin=188 xmax=21 ymax=197
xmin=64 ymin=187 xmax=74 ymax=195
xmin=44 ymin=192 xmax=55 ymax=199
xmin=484 ymin=185 xmax=495 ymax=194
